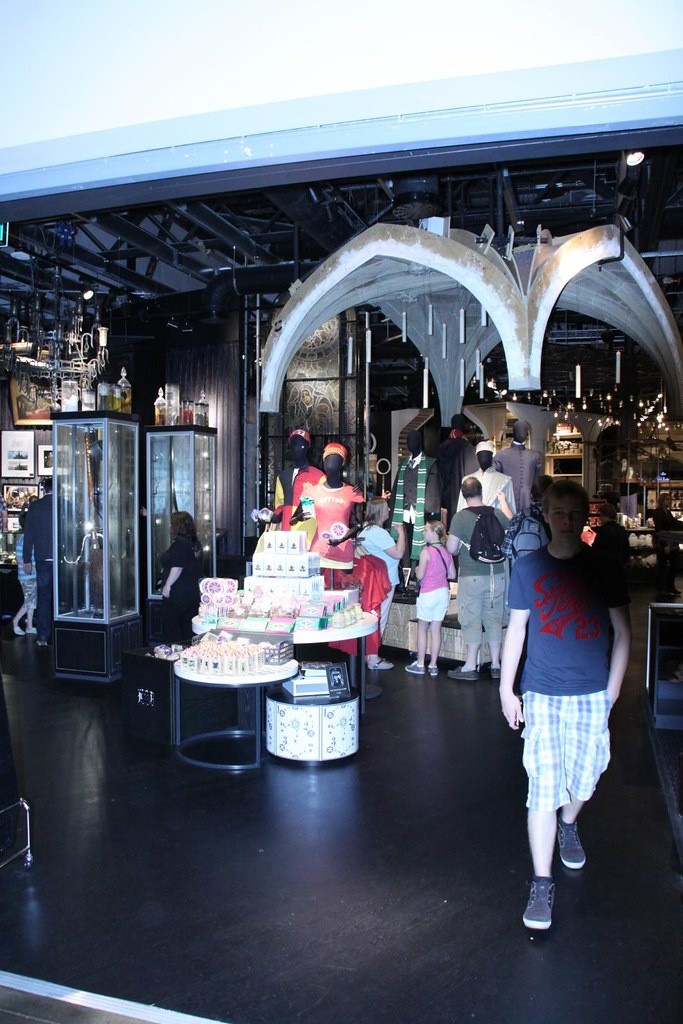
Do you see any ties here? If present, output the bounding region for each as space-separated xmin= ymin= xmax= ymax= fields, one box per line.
xmin=410 ymin=460 xmax=415 ymax=468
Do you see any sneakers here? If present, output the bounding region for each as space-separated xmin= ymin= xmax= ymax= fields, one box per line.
xmin=558 ymin=821 xmax=586 ymax=869
xmin=428 ymin=665 xmax=439 ymax=675
xmin=405 ymin=660 xmax=426 ymax=674
xmin=523 ymin=881 xmax=555 ymax=929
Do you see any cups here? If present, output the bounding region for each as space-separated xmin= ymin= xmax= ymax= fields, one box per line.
xmin=153 ymin=387 xmax=209 ymax=427
xmin=61 ymin=367 xmax=132 ymax=414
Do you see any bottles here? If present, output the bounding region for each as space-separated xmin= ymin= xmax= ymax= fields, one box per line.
xmin=198 ymin=388 xmax=209 ymax=427
xmin=331 ymin=602 xmax=363 ymax=628
xmin=154 ymin=387 xmax=165 ymax=425
xmin=179 ymin=639 xmax=265 ymax=678
xmin=117 ymin=367 xmax=129 ymax=386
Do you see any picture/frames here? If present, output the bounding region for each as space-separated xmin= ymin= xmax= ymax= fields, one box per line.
xmin=2 ymin=483 xmax=39 ymax=513
xmin=36 ymin=443 xmax=73 ymax=476
xmin=9 ymin=369 xmax=58 ymax=429
xmin=1 ymin=431 xmax=35 ymax=477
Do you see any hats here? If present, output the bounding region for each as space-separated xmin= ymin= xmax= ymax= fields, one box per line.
xmin=323 ymin=442 xmax=348 ymax=467
xmin=289 ymin=430 xmax=311 ymax=448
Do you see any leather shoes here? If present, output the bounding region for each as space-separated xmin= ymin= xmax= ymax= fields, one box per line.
xmin=447 ymin=666 xmax=477 ymax=680
xmin=487 ymin=664 xmax=500 ymax=678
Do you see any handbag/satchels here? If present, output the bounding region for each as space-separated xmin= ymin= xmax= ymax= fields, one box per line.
xmin=397 ymin=566 xmax=406 ymax=594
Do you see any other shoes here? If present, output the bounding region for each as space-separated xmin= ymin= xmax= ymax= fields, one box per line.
xmin=35 ymin=638 xmax=47 ymax=646
xmin=666 ymin=588 xmax=681 ymax=594
xmin=9 ymin=624 xmax=25 ymax=635
xmin=26 ymin=628 xmax=37 ymax=634
xmin=655 ymin=584 xmax=666 ymax=591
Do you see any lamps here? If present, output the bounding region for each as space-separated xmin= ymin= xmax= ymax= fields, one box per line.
xmin=78 ymin=275 xmax=93 ymax=299
xmin=616 ymin=148 xmax=645 ymax=233
xmin=96 ymin=326 xmax=110 ymax=347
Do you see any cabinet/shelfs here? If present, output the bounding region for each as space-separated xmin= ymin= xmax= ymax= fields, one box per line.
xmin=546 ymin=432 xmax=584 ymax=487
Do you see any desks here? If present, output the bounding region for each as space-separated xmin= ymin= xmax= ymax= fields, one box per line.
xmin=172 ymin=660 xmax=297 ymax=771
xmin=192 ymin=611 xmax=379 ymax=718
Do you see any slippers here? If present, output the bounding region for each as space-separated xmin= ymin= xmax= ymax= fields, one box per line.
xmin=368 ymin=658 xmax=394 ymax=669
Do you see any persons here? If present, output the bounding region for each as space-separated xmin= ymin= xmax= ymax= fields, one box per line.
xmin=652 ymin=493 xmax=683 ymax=594
xmin=160 ymin=512 xmax=203 ymax=644
xmin=289 ymin=442 xmax=364 ymax=570
xmin=264 ymin=429 xmax=311 ymax=531
xmin=355 ymin=498 xmax=406 ymax=670
xmin=501 ymin=475 xmax=552 ymax=568
xmin=23 ymin=479 xmax=81 ymax=646
xmin=592 ymin=504 xmax=630 ymax=582
xmin=493 ymin=421 xmax=545 ymax=513
xmin=498 ymin=479 xmax=632 ymax=929
xmin=456 ymin=441 xmax=517 ymax=515
xmin=446 ymin=477 xmax=514 ymax=680
xmin=383 ymin=430 xmax=441 ymax=592
xmin=13 ymin=511 xmax=37 ymax=635
xmin=405 ymin=520 xmax=456 ymax=675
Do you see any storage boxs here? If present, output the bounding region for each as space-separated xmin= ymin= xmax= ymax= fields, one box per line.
xmin=121 ymin=641 xmax=237 ymax=741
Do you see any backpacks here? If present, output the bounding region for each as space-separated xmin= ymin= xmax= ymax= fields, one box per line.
xmin=459 ymin=507 xmax=506 ymax=564
xmin=512 ymin=507 xmax=550 ymax=558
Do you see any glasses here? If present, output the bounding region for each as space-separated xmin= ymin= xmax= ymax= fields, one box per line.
xmin=665 ymin=499 xmax=672 ymax=500
